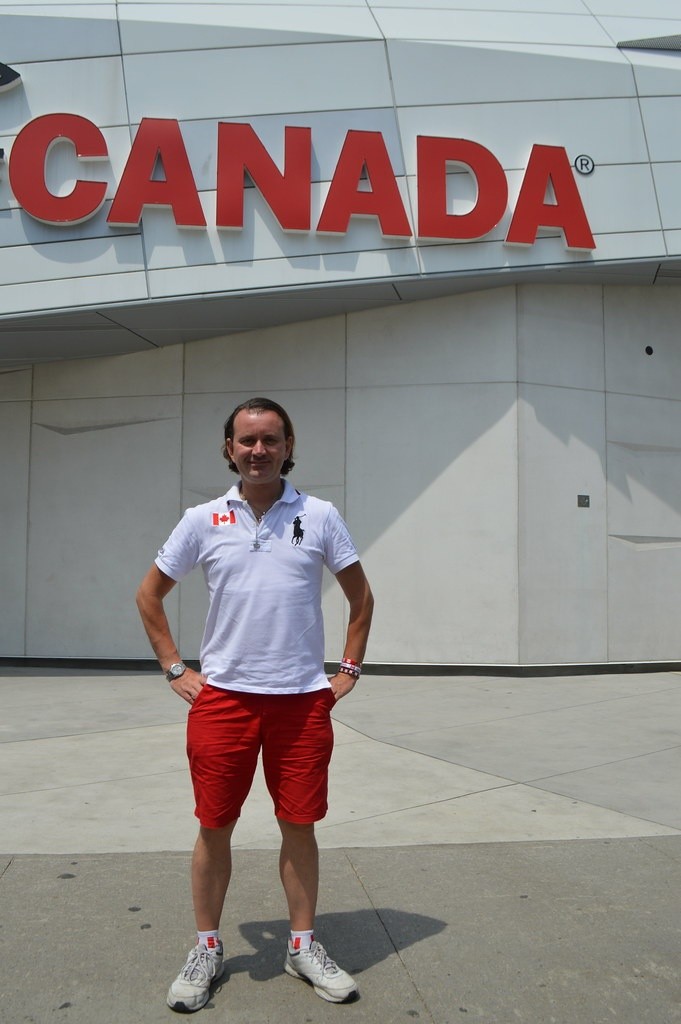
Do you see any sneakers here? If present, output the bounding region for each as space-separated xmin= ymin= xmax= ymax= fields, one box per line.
xmin=166 ymin=940 xmax=226 ymax=1012
xmin=282 ymin=935 xmax=358 ymax=1003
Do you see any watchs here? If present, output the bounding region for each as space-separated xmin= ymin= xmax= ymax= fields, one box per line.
xmin=165 ymin=662 xmax=187 ymax=683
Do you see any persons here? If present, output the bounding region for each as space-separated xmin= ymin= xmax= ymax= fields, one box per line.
xmin=135 ymin=397 xmax=375 ymax=1011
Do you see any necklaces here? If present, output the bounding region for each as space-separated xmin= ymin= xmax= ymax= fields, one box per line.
xmin=249 ymin=502 xmax=265 ymax=523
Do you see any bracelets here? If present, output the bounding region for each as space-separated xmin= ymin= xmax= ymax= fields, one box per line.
xmin=338 ymin=658 xmax=362 ymax=678
